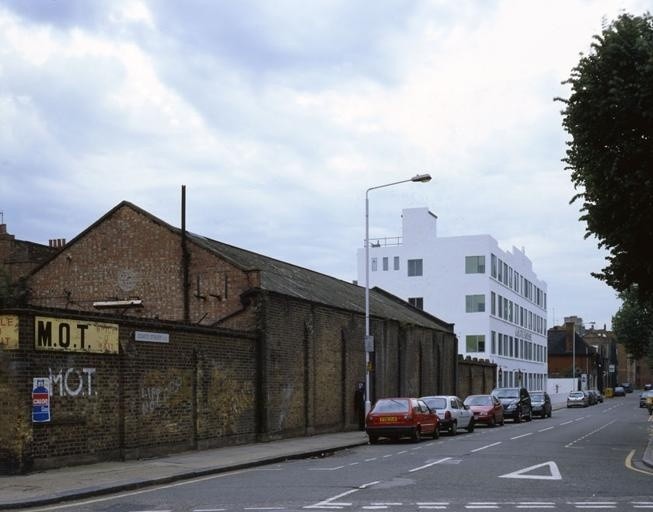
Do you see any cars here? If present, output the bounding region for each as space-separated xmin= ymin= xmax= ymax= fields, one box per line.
xmin=568 ymin=390 xmax=603 ymax=408
xmin=640 ymin=390 xmax=653 ymax=408
xmin=463 ymin=395 xmax=504 ymax=428
xmin=528 ymin=391 xmax=552 ymax=419
xmin=421 ymin=396 xmax=475 ymax=436
xmin=365 ymin=398 xmax=441 ymax=444
xmin=614 ymin=383 xmax=634 ymax=396
xmin=644 ymin=384 xmax=652 ymax=390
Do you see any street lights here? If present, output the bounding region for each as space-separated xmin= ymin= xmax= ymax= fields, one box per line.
xmin=365 ymin=174 xmax=432 ymax=429
xmin=572 ymin=322 xmax=607 ymax=391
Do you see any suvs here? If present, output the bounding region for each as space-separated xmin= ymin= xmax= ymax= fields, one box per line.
xmin=491 ymin=387 xmax=532 ymax=423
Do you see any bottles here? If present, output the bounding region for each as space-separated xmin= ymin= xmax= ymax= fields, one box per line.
xmin=31 ymin=379 xmax=50 ymax=422
